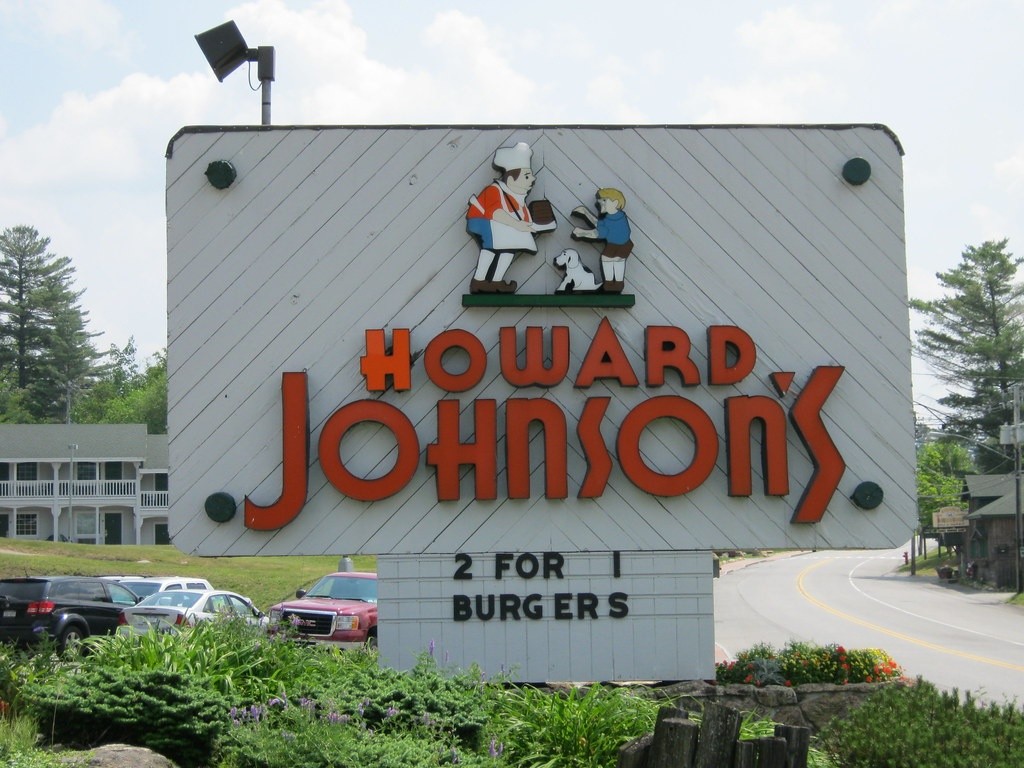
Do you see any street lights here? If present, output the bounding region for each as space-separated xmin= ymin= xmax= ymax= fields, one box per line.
xmin=928 ymin=429 xmax=1024 ymax=594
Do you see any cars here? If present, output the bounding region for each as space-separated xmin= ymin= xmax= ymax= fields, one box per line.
xmin=117 ymin=591 xmax=277 ymax=640
xmin=266 ymin=571 xmax=378 ymax=653
xmin=1 ymin=575 xmax=145 ymax=662
xmin=101 ymin=575 xmax=215 ymax=602
